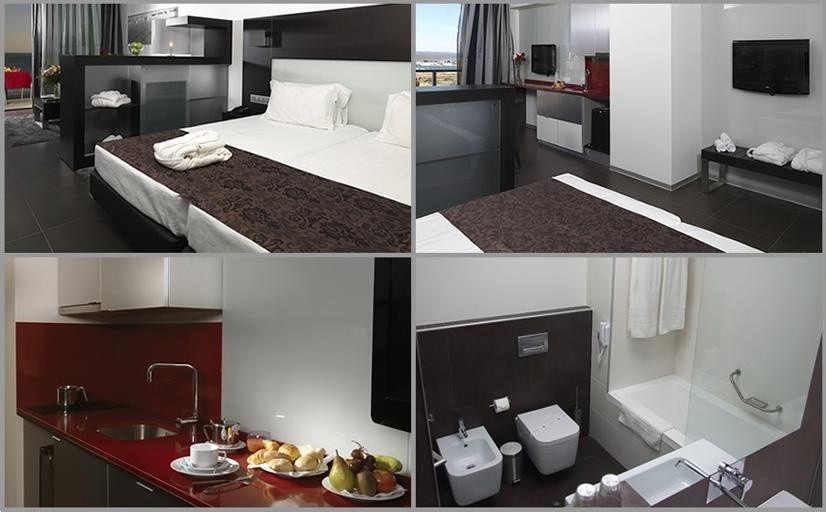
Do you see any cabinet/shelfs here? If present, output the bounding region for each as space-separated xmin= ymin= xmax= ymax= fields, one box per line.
xmin=57 ymin=53 xmax=232 ymax=174
xmin=535 ymin=87 xmax=594 ymax=155
xmin=58 ymin=255 xmax=99 ymax=316
xmin=584 ymin=56 xmax=609 ymax=101
xmin=108 ymin=462 xmax=160 ymax=507
xmin=160 ymin=487 xmax=193 ymax=505
xmin=101 ymin=256 xmax=223 ymax=312
xmin=37 ymin=429 xmax=108 ymax=507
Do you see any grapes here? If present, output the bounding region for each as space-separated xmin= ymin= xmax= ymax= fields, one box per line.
xmin=349 ymin=447 xmax=376 ymax=474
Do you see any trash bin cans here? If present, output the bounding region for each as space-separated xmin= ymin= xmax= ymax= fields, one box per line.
xmin=500 ymin=441 xmax=524 ymax=485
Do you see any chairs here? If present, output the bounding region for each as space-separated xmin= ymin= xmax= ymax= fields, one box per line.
xmin=3 ymin=70 xmax=33 ymax=100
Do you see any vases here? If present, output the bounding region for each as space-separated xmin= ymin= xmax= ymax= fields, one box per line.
xmin=514 ymin=62 xmax=524 ymax=82
xmin=53 ymin=82 xmax=61 ymax=98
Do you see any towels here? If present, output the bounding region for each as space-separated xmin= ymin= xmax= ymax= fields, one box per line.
xmin=657 ymin=256 xmax=691 ymax=337
xmin=712 ymin=138 xmax=726 ymax=153
xmin=627 ymin=256 xmax=664 ymax=340
xmin=720 ymin=132 xmax=737 ymax=153
xmin=617 ymin=394 xmax=675 ymax=453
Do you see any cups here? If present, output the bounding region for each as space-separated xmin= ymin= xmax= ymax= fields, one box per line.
xmin=571 ymin=474 xmax=622 ymax=506
xmin=203 ymin=419 xmax=240 ymax=448
xmin=189 ymin=442 xmax=227 ymax=467
xmin=247 ymin=430 xmax=272 ymax=453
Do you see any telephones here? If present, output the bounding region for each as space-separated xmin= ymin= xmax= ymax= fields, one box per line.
xmin=232 ymin=106 xmax=250 ymax=114
xmin=597 ymin=322 xmax=610 ymax=349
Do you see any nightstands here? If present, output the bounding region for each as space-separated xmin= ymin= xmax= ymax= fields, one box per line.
xmin=222 ymin=102 xmax=266 ymax=120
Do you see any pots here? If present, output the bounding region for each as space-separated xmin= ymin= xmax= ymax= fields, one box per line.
xmin=55 ymin=384 xmax=88 ymax=410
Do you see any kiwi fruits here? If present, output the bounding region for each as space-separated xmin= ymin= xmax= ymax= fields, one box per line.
xmin=355 ymin=471 xmax=378 ymax=496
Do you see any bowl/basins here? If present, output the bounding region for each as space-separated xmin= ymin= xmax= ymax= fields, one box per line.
xmin=128 ymin=45 xmax=145 ymax=55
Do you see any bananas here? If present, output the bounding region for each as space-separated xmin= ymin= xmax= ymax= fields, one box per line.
xmin=371 ymin=454 xmax=402 ymax=473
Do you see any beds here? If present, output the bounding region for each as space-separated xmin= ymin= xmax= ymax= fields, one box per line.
xmin=416 ymin=172 xmax=769 ymax=253
xmin=89 ymin=57 xmax=412 ymax=253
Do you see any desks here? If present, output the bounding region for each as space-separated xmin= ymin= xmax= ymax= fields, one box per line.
xmin=700 ymin=145 xmax=822 ymax=209
xmin=32 ymin=96 xmax=60 ymax=128
xmin=502 ymin=79 xmax=585 ymax=124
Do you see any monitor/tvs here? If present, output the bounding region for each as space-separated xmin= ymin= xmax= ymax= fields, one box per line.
xmin=530 ymin=44 xmax=557 ymax=75
xmin=731 ymin=38 xmax=812 ymax=97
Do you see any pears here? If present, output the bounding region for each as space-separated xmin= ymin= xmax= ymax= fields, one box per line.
xmin=328 ymin=455 xmax=355 ymax=492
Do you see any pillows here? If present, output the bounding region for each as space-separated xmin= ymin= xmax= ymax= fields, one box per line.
xmin=375 ymin=93 xmax=411 ymax=148
xmin=398 ymin=89 xmax=412 ymax=100
xmin=265 ymin=79 xmax=343 ymax=131
xmin=281 ymin=80 xmax=353 ymax=129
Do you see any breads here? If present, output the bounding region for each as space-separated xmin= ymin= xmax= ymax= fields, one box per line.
xmin=247 ymin=439 xmax=326 ymax=472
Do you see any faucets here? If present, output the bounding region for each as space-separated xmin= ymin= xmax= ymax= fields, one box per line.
xmin=143 ymin=361 xmax=201 ymax=436
xmin=674 ymin=455 xmax=753 ymax=507
xmin=458 ymin=420 xmax=471 ymax=440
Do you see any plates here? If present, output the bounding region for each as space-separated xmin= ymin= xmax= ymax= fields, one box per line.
xmin=170 ymin=456 xmax=240 ymax=476
xmin=180 ymin=457 xmax=230 ymax=470
xmin=209 ymin=440 xmax=246 ymax=453
xmin=257 ymin=469 xmax=322 ymax=493
xmin=321 ymin=491 xmax=402 ymax=506
xmin=322 ymin=476 xmax=405 ymax=500
xmin=247 ymin=460 xmax=329 ymax=478
xmin=169 ymin=471 xmax=239 ymax=488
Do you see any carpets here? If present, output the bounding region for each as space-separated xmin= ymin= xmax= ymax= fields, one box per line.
xmin=2 ymin=114 xmax=61 ymax=149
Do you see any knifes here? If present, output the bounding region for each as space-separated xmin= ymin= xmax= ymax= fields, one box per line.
xmin=204 ymin=473 xmax=253 ymax=493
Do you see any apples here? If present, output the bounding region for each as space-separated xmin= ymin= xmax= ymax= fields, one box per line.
xmin=129 ymin=43 xmax=143 ymax=54
xmin=371 ymin=469 xmax=396 ymax=493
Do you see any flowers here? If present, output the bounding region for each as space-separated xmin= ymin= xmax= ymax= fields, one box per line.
xmin=512 ymin=53 xmax=528 ymax=66
xmin=36 ymin=62 xmax=61 ymax=88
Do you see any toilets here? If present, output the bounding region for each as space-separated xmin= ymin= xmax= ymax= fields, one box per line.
xmin=514 ymin=403 xmax=581 ymax=477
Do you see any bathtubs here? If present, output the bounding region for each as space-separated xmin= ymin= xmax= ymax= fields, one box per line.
xmin=607 ymin=373 xmax=786 ymax=468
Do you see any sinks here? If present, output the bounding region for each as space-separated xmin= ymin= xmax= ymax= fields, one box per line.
xmin=96 ymin=424 xmax=177 ymax=442
xmin=437 ymin=427 xmax=504 ymax=506
xmin=622 ymin=458 xmax=709 ymax=507
